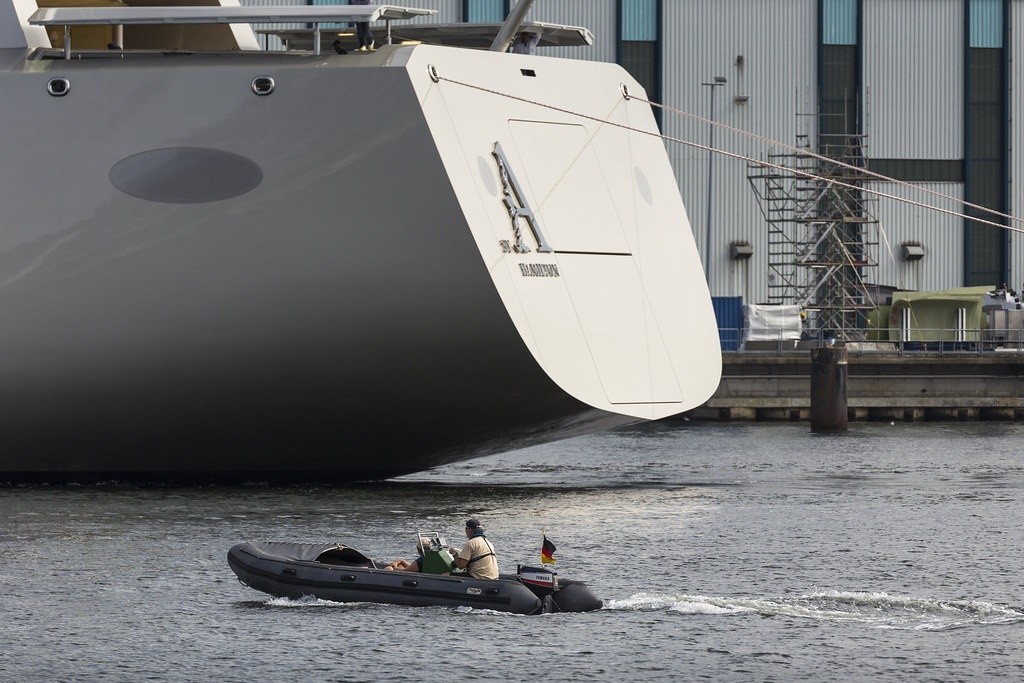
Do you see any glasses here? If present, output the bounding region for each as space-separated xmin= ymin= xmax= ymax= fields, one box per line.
xmin=466 ymin=527 xmax=470 ymax=530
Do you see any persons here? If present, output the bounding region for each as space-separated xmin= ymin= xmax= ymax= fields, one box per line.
xmin=107 ymin=42 xmax=122 ymax=58
xmin=448 ymin=518 xmax=498 ymax=580
xmin=350 ymin=0 xmax=374 ymax=51
xmin=333 ymin=40 xmax=348 ymax=54
xmin=384 ymin=539 xmax=432 ymax=572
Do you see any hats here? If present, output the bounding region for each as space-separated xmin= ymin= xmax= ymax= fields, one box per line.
xmin=466 ymin=519 xmax=484 ymax=532
xmin=417 ymin=538 xmax=433 ymax=549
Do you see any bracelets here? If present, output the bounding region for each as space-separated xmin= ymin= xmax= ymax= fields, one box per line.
xmin=453 ymin=552 xmax=458 ymax=558
xmin=392 ymin=564 xmax=397 ymax=568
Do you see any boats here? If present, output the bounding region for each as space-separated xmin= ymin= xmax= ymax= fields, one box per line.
xmin=0 ymin=1 xmax=726 ymax=483
xmin=226 ymin=540 xmax=603 ymax=617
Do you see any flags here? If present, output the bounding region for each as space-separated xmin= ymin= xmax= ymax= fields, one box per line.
xmin=541 ymin=537 xmax=557 ymax=566
